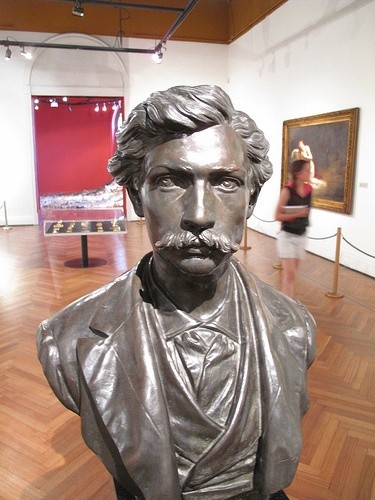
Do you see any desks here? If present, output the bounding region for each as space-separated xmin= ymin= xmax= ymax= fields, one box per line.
xmin=44 ymin=219 xmax=127 ymax=268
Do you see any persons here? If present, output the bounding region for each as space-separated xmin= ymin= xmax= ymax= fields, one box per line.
xmin=35 ymin=83 xmax=319 ymax=500
xmin=274 ymin=141 xmax=315 ymax=298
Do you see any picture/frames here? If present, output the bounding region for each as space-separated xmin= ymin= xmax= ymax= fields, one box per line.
xmin=280 ymin=108 xmax=360 ymax=215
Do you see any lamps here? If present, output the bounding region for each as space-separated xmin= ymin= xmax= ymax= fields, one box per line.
xmin=34 ymin=95 xmax=122 ymax=114
xmin=5 ymin=45 xmax=11 ymax=61
xmin=154 ymin=48 xmax=163 ymax=64
xmin=72 ymin=1 xmax=84 ymax=18
xmin=20 ymin=45 xmax=32 ymax=59
